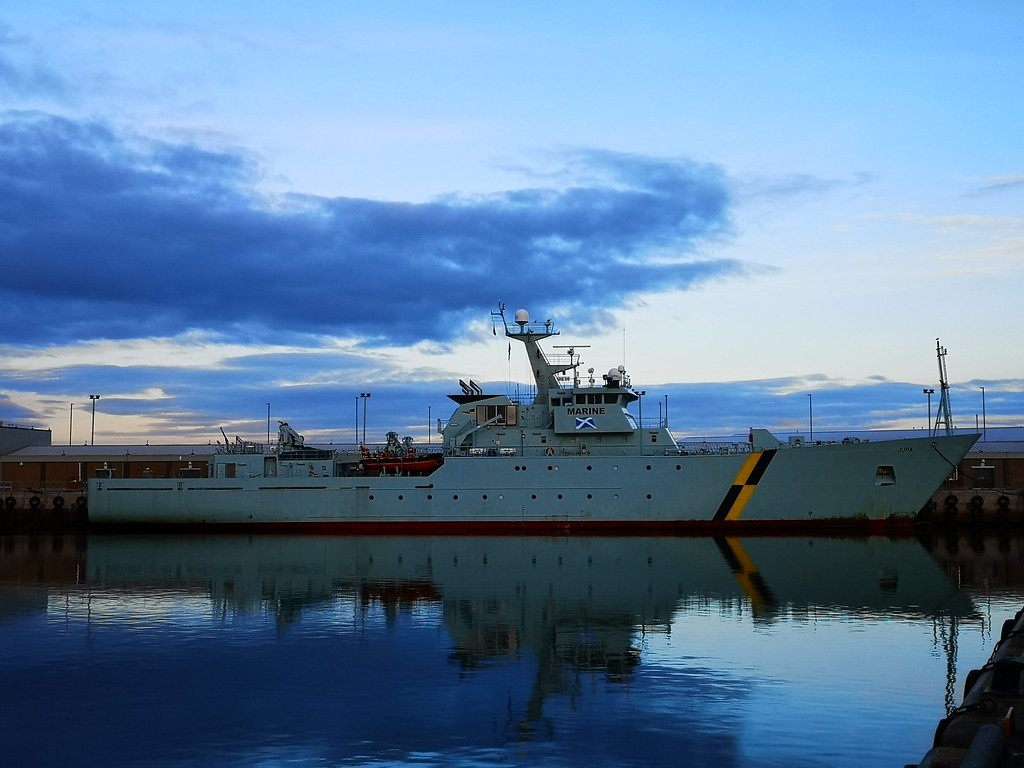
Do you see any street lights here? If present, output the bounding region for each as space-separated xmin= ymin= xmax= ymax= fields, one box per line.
xmin=664 ymin=394 xmax=668 ymax=421
xmin=922 ymin=388 xmax=934 ymax=438
xmin=658 ymin=401 xmax=662 ymax=428
xmin=68 ymin=402 xmax=76 ymax=447
xmin=353 ymin=395 xmax=360 ymax=445
xmin=359 ymin=393 xmax=371 ymax=445
xmin=807 ymin=393 xmax=813 ymax=444
xmin=428 ymin=405 xmax=432 ymax=444
xmin=265 ymin=401 xmax=271 ymax=445
xmin=980 ymin=385 xmax=986 ymax=443
xmin=89 ymin=394 xmax=100 ymax=447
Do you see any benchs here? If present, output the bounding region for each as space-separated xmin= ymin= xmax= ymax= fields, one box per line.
xmin=0 ymin=481 xmax=13 ymax=492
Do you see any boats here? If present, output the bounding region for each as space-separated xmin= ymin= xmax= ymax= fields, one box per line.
xmin=79 ymin=523 xmax=986 ymax=721
xmin=361 ymin=446 xmax=439 ymax=474
xmin=89 ymin=296 xmax=985 ymax=533
